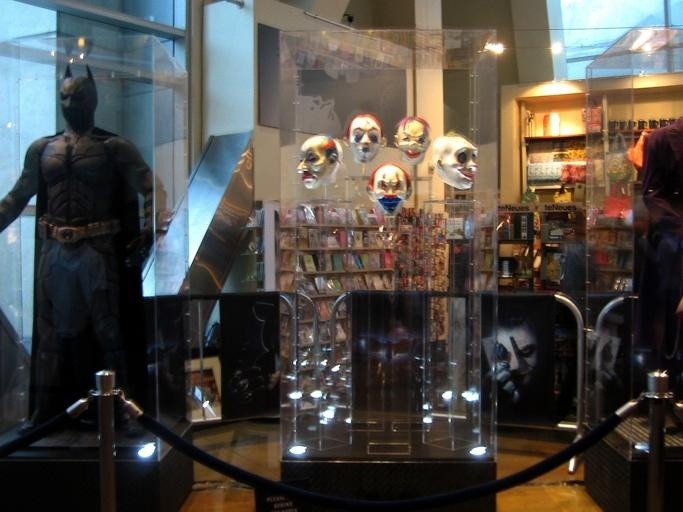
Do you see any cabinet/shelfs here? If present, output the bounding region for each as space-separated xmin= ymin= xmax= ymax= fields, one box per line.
xmin=497 ymin=87 xmax=683 ymax=292
xmin=277 ymin=207 xmax=399 ymax=371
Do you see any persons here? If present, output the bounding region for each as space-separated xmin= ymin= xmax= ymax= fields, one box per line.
xmin=642 ymin=114 xmax=683 ymax=347
xmin=0 ymin=62 xmax=173 ymax=435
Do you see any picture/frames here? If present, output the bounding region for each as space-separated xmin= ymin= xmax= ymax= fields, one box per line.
xmin=182 ymin=356 xmax=223 ymax=423
